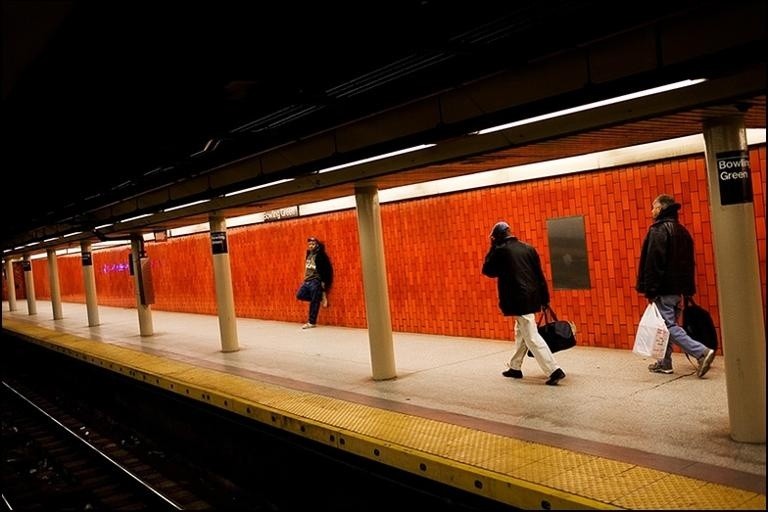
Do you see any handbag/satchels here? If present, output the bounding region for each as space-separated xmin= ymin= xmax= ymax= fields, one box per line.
xmin=528 ymin=302 xmax=576 ymax=357
xmin=682 ymin=298 xmax=718 ymax=357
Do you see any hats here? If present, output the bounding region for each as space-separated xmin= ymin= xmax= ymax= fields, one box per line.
xmin=491 ymin=223 xmax=510 ymax=237
xmin=308 ymin=237 xmax=319 ymax=243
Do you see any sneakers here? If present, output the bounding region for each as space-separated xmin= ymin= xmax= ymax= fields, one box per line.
xmin=302 ymin=322 xmax=316 ymax=329
xmin=322 ymin=293 xmax=328 ymax=307
xmin=697 ymin=349 xmax=716 ymax=378
xmin=647 ymin=364 xmax=674 ymax=374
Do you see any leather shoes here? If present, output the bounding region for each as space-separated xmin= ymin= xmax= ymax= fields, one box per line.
xmin=504 ymin=369 xmax=524 ymax=379
xmin=545 ymin=369 xmax=566 ymax=384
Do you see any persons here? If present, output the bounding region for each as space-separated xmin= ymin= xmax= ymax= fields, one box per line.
xmin=633 ymin=194 xmax=714 ymax=379
xmin=295 ymin=237 xmax=335 ymax=329
xmin=480 ymin=222 xmax=565 ymax=385
